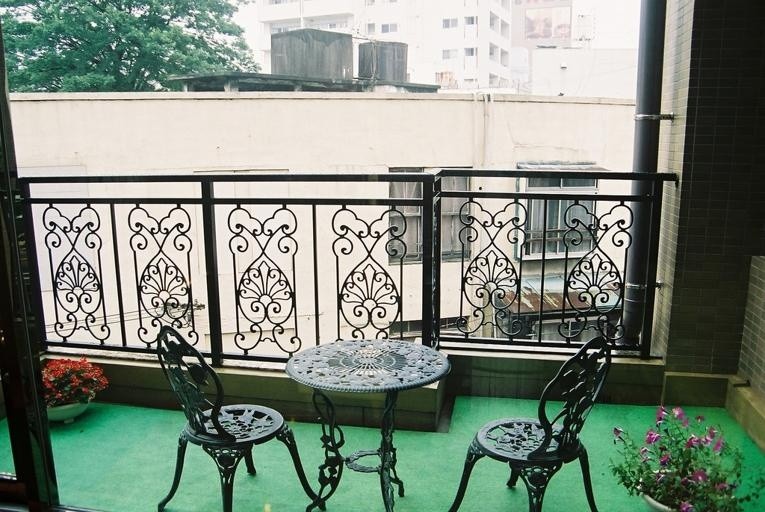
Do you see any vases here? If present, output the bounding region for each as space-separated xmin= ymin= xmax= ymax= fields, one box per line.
xmin=637 ymin=468 xmax=694 ymax=511
xmin=47 ymin=400 xmax=92 ymax=424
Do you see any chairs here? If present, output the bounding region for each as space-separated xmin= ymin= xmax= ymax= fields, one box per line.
xmin=449 ymin=336 xmax=612 ymax=512
xmin=155 ymin=325 xmax=328 ymax=512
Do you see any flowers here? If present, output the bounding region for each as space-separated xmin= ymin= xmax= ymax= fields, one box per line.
xmin=612 ymin=405 xmax=765 ymax=510
xmin=41 ymin=357 xmax=109 ymax=408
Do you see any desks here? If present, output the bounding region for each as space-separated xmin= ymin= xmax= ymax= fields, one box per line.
xmin=285 ymin=338 xmax=451 ymax=512
